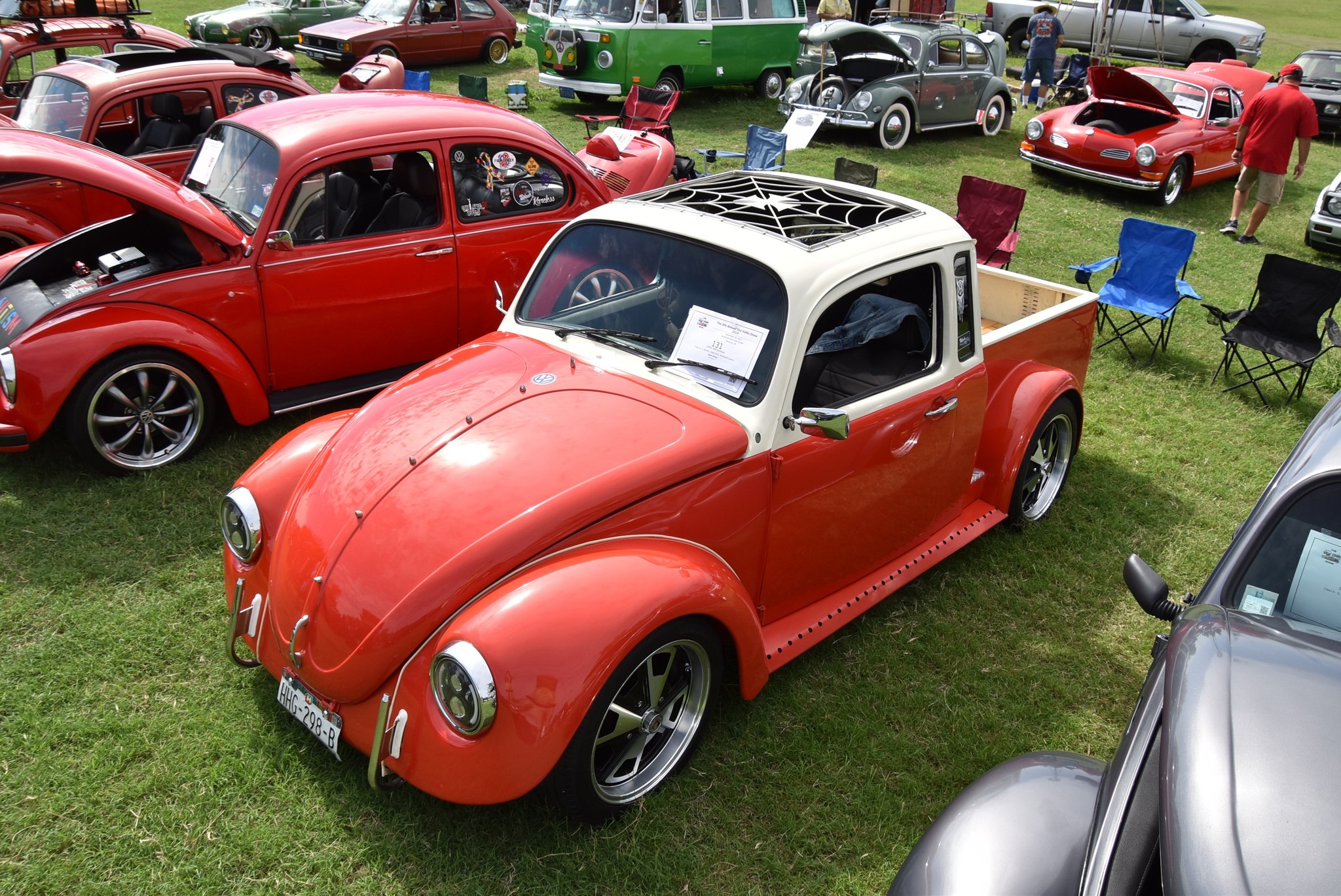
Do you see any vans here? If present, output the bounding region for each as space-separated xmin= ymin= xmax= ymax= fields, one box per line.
xmin=525 ymin=0 xmax=809 ymax=123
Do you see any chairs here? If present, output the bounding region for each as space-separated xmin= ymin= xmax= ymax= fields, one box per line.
xmin=1200 ymin=251 xmax=1341 ymax=411
xmin=1046 ymin=52 xmax=1098 ymax=106
xmin=1067 ymin=217 xmax=1203 ymax=368
xmin=458 ymin=73 xmax=490 ymax=102
xmin=414 ymin=4 xmax=432 ymax=24
xmin=832 ymin=158 xmax=880 ymax=190
xmin=951 ymin=174 xmax=1028 ymax=270
xmin=320 ymin=155 xmax=377 ymax=238
xmin=575 ymin=83 xmax=682 ymax=148
xmin=192 ymin=104 xmax=214 ymax=144
xmin=365 ymin=152 xmax=434 ymax=236
xmin=798 ymin=290 xmax=927 ymax=410
xmin=1006 ymin=49 xmax=1069 ymax=81
xmin=693 ymin=122 xmax=789 ymax=182
xmin=656 ymin=253 xmax=778 ymax=361
xmin=404 ymin=68 xmax=432 ymax=91
xmin=435 ymin=4 xmax=454 ymax=22
xmin=126 ymin=93 xmax=192 ymax=156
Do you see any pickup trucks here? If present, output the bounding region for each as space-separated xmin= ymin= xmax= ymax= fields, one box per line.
xmin=222 ymin=168 xmax=1100 ymax=823
xmin=980 ymin=0 xmax=1268 ymax=70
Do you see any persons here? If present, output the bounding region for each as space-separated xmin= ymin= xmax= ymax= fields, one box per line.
xmin=816 ymin=0 xmax=852 ymax=23
xmin=1020 ymin=2 xmax=1065 ymax=112
xmin=1219 ymin=64 xmax=1319 ymax=245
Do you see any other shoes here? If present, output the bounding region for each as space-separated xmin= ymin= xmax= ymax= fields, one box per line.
xmin=1021 ymin=105 xmax=1027 ymax=109
xmin=1219 ymin=219 xmax=1238 ymax=234
xmin=1238 ymin=234 xmax=1262 ymax=245
xmin=1034 ymin=106 xmax=1042 ymax=111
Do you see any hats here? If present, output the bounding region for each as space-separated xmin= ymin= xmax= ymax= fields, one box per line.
xmin=1273 ymin=63 xmax=1303 ymax=82
xmin=1033 ymin=2 xmax=1057 ymax=16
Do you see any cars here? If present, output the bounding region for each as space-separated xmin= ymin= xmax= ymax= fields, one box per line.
xmin=294 ymin=0 xmax=522 ymax=72
xmin=0 ymin=19 xmax=693 ymax=483
xmin=890 ymin=389 xmax=1341 ymax=896
xmin=1260 ymin=48 xmax=1341 ymax=135
xmin=776 ymin=9 xmax=1012 ymax=150
xmin=184 ymin=1 xmax=394 ymax=53
xmin=1019 ymin=59 xmax=1276 ymax=207
